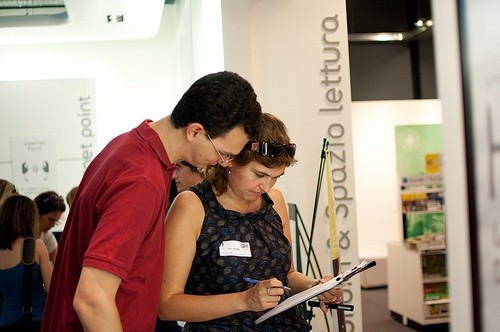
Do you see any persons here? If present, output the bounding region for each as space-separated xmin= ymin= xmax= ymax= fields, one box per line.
xmin=0 ymin=194 xmax=53 ymax=332
xmin=155 ymin=113 xmax=344 ymax=332
xmin=172 ymin=160 xmax=203 ymax=192
xmin=67 ymin=185 xmax=78 ymax=212
xmin=37 ymin=70 xmax=263 ymax=332
xmin=0 ymin=178 xmax=17 ymax=209
xmin=32 ymin=190 xmax=66 ymax=267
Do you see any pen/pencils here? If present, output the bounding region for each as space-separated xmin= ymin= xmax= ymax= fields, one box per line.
xmin=243 ymin=277 xmax=291 ymax=290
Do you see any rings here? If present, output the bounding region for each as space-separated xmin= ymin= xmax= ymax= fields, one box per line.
xmin=267 ymin=289 xmax=270 ymax=295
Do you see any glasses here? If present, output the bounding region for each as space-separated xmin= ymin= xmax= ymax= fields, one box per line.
xmin=207 ymin=134 xmax=235 ymax=164
xmin=244 ymin=139 xmax=297 ymax=157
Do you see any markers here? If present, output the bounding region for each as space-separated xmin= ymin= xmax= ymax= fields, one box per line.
xmin=308 ymin=301 xmax=354 ymax=311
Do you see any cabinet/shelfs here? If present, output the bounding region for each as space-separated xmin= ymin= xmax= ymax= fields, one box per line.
xmin=387 ymin=239 xmax=447 ymax=325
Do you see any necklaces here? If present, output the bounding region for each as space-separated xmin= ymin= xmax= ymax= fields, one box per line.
xmin=223 ymin=191 xmax=252 ymax=216
